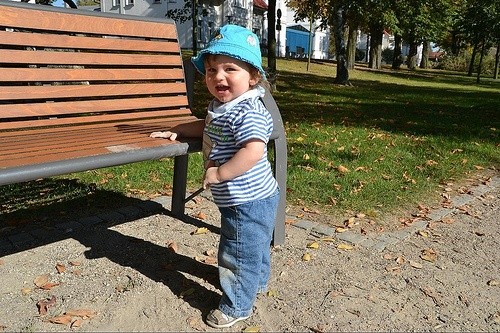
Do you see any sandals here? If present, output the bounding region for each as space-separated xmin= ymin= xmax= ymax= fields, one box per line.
xmin=200 ymin=304 xmax=252 ymax=328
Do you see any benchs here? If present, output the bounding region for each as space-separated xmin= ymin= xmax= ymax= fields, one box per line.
xmin=0 ymin=0 xmax=288 ymax=248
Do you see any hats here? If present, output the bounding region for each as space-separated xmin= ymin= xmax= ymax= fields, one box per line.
xmin=190 ymin=24 xmax=267 ymax=79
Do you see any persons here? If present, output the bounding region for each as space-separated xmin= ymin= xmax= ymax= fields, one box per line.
xmin=148 ymin=24 xmax=282 ymax=329
